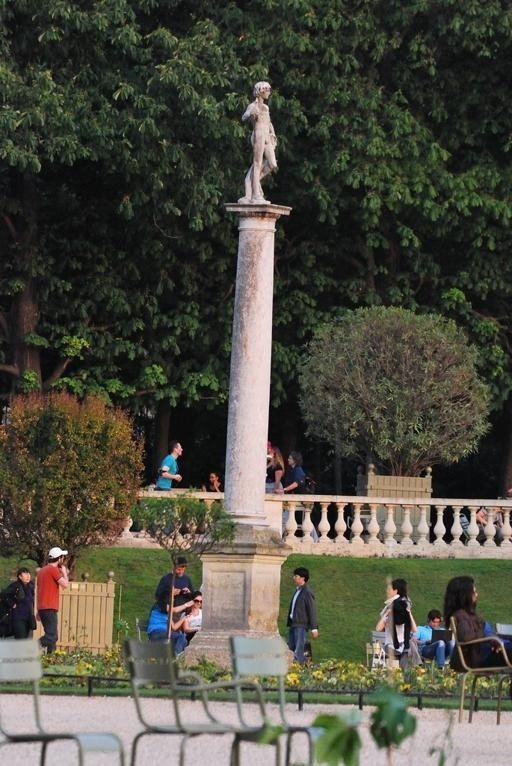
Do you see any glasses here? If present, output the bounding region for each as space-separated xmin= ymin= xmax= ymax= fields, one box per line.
xmin=194 ymin=599 xmax=203 ymax=604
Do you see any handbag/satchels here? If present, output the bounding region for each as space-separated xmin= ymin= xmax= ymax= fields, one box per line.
xmin=1 ymin=608 xmax=12 ymax=626
xmin=303 ymin=641 xmax=311 ymax=663
xmin=304 ymin=477 xmax=317 ymax=494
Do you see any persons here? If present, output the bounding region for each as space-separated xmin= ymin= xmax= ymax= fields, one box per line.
xmin=286 ymin=566 xmax=320 ymax=666
xmin=145 ymin=590 xmax=195 ymax=659
xmin=201 ymin=470 xmax=224 ymax=492
xmin=182 ymin=590 xmax=202 ymax=645
xmin=0 ymin=566 xmax=35 ymax=638
xmin=475 ymin=505 xmax=505 ymax=545
xmin=35 ymin=546 xmax=69 ymax=655
xmin=150 ymin=439 xmax=184 ymax=534
xmin=154 ymin=557 xmax=193 ymax=630
xmin=376 ymin=605 xmax=424 ymax=668
xmin=412 ymin=608 xmax=456 ymax=669
xmin=443 ymin=575 xmax=512 ymax=674
xmin=276 ymin=449 xmax=306 ymax=537
xmin=240 ymin=81 xmax=279 ymax=203
xmin=266 ymin=445 xmax=285 ymax=496
xmin=375 ymin=577 xmax=410 ymax=672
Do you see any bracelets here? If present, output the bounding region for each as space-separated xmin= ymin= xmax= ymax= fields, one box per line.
xmin=283 ymin=487 xmax=287 ymax=494
xmin=184 ymin=602 xmax=188 ymax=608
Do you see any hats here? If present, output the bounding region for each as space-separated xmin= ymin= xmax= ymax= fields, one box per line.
xmin=48 ymin=546 xmax=68 ymax=560
